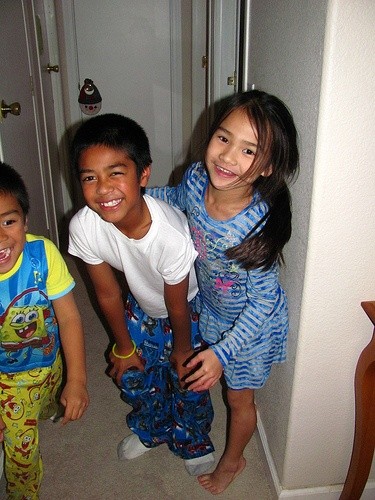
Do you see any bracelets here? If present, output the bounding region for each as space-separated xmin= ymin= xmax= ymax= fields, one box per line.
xmin=112 ymin=339 xmax=136 ymax=360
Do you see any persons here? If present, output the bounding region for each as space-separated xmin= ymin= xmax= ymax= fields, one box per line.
xmin=66 ymin=114 xmax=217 ymax=477
xmin=0 ymin=161 xmax=90 ymax=500
xmin=145 ymin=89 xmax=301 ymax=496
